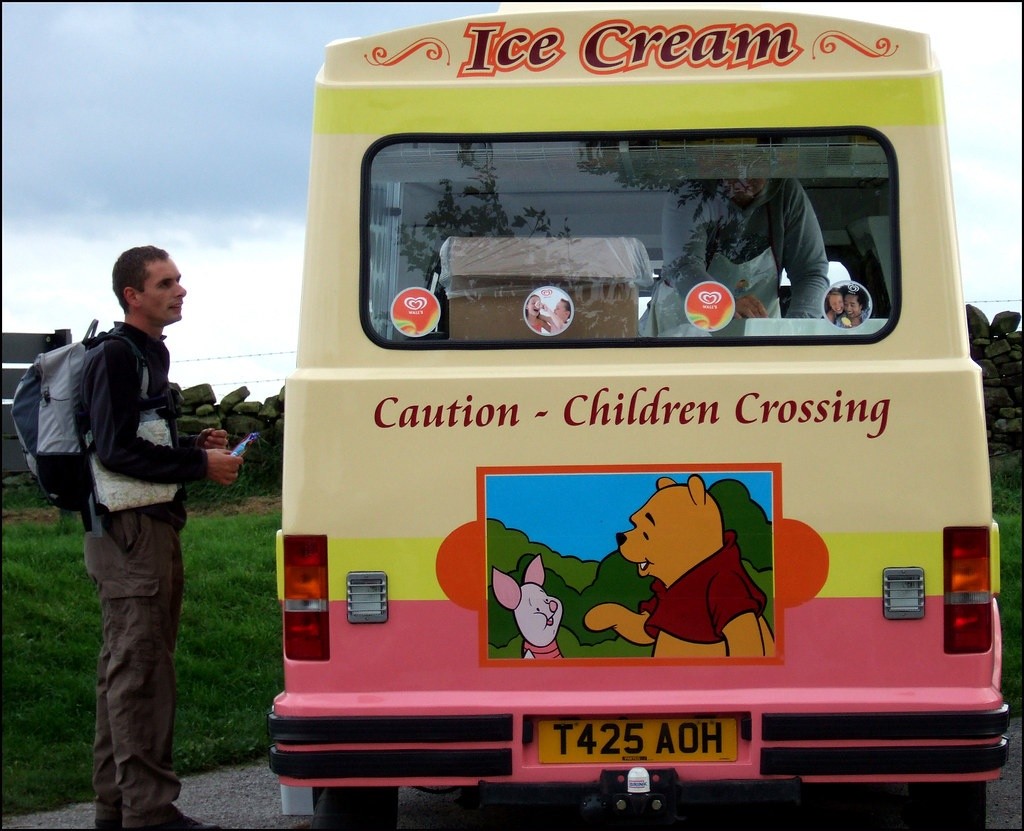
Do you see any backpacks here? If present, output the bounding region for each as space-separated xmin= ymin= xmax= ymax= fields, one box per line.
xmin=10 ymin=320 xmax=148 ymax=537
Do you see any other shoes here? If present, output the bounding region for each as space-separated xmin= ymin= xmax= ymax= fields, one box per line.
xmin=123 ymin=815 xmax=221 ymax=831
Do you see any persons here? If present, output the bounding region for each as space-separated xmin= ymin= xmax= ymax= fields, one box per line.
xmin=527 ymin=295 xmax=571 ymax=334
xmin=83 ymin=247 xmax=244 ymax=831
xmin=639 ymin=135 xmax=828 ymax=340
xmin=826 ymin=290 xmax=869 ymax=328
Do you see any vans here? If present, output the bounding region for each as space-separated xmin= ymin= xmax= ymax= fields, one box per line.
xmin=263 ymin=10 xmax=999 ymax=831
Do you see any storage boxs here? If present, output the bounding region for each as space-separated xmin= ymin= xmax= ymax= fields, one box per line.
xmin=439 ymin=235 xmax=655 ymax=341
